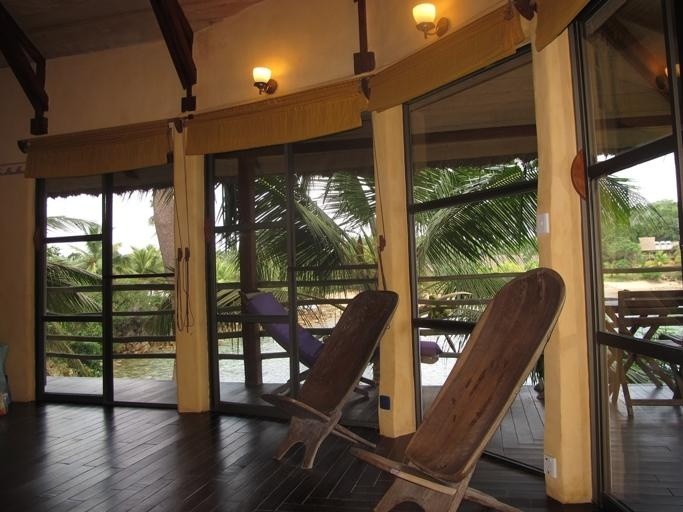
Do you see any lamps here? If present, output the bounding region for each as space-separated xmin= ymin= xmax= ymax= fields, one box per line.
xmin=249 ymin=65 xmax=280 ymax=95
xmin=408 ymin=3 xmax=451 ymax=44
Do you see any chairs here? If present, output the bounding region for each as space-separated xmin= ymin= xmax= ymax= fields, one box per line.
xmin=602 ymin=285 xmax=683 ymax=423
xmin=239 ymin=288 xmax=445 ymax=417
